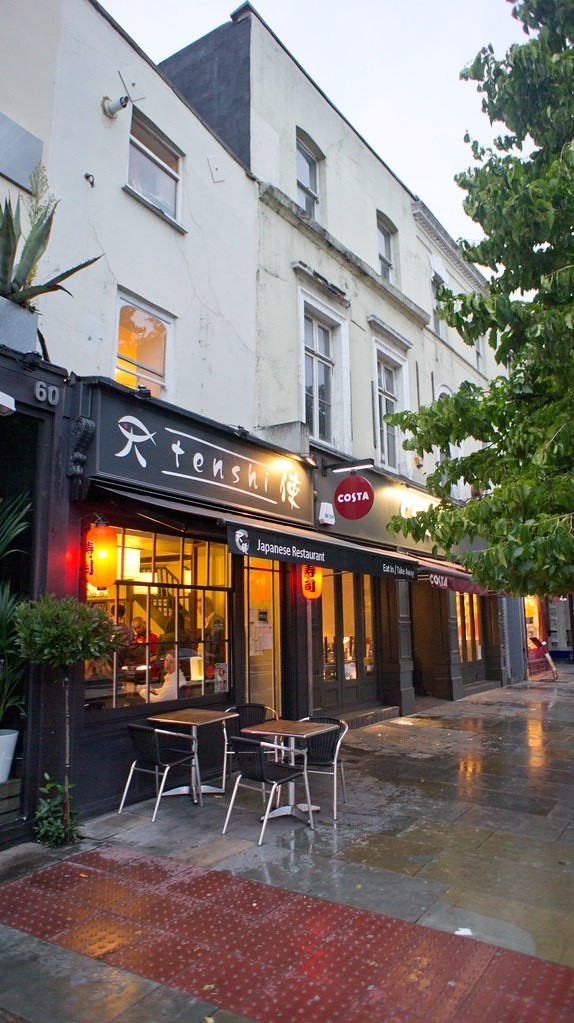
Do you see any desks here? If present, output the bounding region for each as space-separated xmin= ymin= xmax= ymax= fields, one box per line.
xmin=84 ymin=680 xmax=215 ymax=703
xmin=147 ymin=708 xmax=238 ymax=797
xmin=241 ymin=719 xmax=339 ymax=831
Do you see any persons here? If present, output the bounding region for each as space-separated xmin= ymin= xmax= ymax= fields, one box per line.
xmin=135 ymin=650 xmax=187 ymax=703
xmin=102 ymin=596 xmax=227 ymax=670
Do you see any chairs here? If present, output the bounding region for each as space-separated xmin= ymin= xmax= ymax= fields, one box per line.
xmin=177 ymin=685 xmax=192 ymax=699
xmin=281 ymin=716 xmax=348 ymax=819
xmin=119 ymin=723 xmax=203 ymax=822
xmin=223 ymin=737 xmax=313 ymax=846
xmin=223 ymin=703 xmax=279 ymax=799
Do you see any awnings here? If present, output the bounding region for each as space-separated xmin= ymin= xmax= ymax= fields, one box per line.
xmin=96 ymin=481 xmax=419 ymax=580
xmin=404 ymin=554 xmax=574 ymax=604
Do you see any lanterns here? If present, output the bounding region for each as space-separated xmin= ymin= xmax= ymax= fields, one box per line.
xmin=301 ymin=562 xmax=322 ymax=600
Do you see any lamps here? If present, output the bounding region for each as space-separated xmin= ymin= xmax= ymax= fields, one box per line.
xmin=85 ymin=518 xmax=118 ymax=590
xmin=302 ymin=565 xmax=324 ymax=600
xmin=322 ymin=456 xmax=373 ymax=476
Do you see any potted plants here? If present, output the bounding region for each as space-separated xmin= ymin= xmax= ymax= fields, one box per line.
xmin=0 ymin=185 xmax=105 ymax=353
xmin=0 ymin=491 xmax=128 ymax=782
